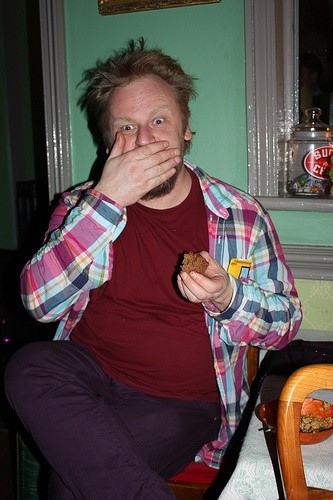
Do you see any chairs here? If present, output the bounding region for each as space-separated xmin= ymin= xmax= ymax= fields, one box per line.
xmin=278 ymin=364 xmax=333 ymax=500
xmin=168 ymin=344 xmax=260 ymax=500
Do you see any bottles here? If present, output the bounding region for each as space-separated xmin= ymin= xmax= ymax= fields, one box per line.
xmin=288 ymin=107 xmax=333 ymax=199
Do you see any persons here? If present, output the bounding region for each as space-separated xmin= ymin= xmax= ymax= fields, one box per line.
xmin=3 ymin=36 xmax=303 ymax=500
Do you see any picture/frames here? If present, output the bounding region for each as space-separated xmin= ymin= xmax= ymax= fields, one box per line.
xmin=98 ymin=0 xmax=221 ymax=18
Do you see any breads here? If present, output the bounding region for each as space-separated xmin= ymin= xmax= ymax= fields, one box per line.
xmin=179 ymin=252 xmax=209 ymax=274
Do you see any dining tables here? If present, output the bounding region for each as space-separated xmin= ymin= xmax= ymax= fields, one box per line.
xmin=221 ymin=373 xmax=333 ymax=500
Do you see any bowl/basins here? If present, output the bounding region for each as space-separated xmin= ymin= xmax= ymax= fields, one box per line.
xmin=258 ymin=396 xmax=333 ymax=446
